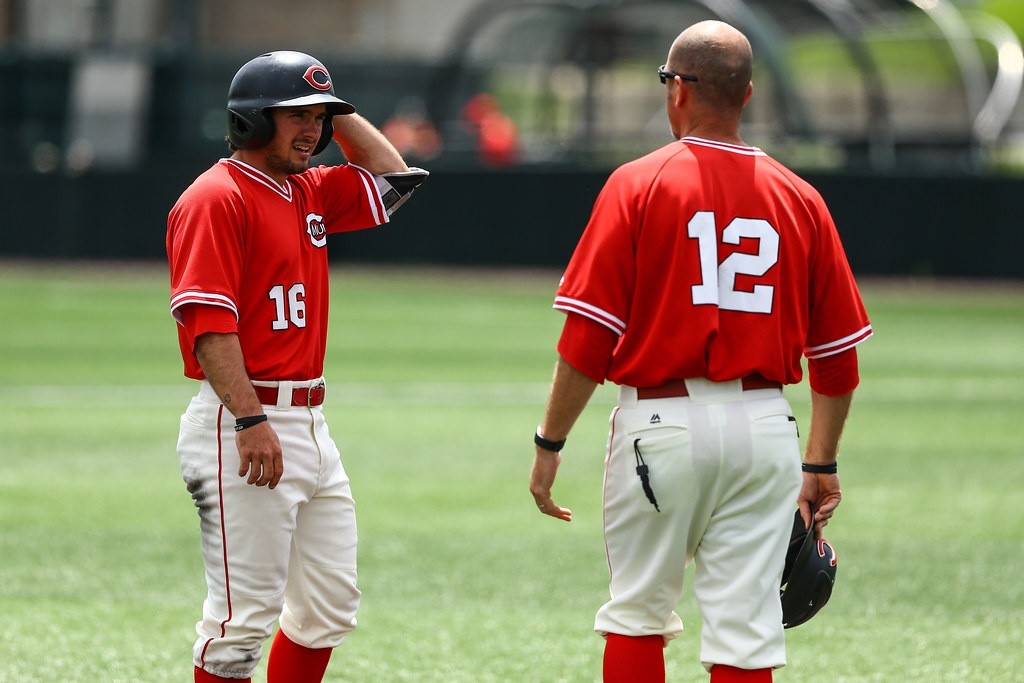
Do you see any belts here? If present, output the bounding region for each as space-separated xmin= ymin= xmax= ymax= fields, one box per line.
xmin=252 ymin=384 xmax=324 ymax=408
xmin=637 ymin=372 xmax=783 ymax=399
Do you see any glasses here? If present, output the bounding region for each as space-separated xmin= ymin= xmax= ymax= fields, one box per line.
xmin=658 ymin=65 xmax=698 ymax=85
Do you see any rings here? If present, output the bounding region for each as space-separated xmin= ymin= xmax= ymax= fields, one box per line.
xmin=539 ymin=504 xmax=546 ymax=507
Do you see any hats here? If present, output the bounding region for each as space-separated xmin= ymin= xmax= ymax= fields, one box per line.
xmin=779 ymin=504 xmax=837 ymax=629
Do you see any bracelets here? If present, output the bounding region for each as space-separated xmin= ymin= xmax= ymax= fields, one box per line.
xmin=801 ymin=462 xmax=838 ymax=468
xmin=534 ymin=426 xmax=566 ymax=453
xmin=235 ymin=415 xmax=267 ymax=424
xmin=801 ymin=465 xmax=837 ymax=474
xmin=234 ymin=422 xmax=263 ymax=432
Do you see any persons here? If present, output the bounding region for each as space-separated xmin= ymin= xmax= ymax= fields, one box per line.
xmin=528 ymin=20 xmax=873 ymax=683
xmin=166 ymin=51 xmax=432 ymax=683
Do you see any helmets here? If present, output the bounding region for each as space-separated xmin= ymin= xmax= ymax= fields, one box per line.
xmin=227 ymin=51 xmax=356 ymax=157
